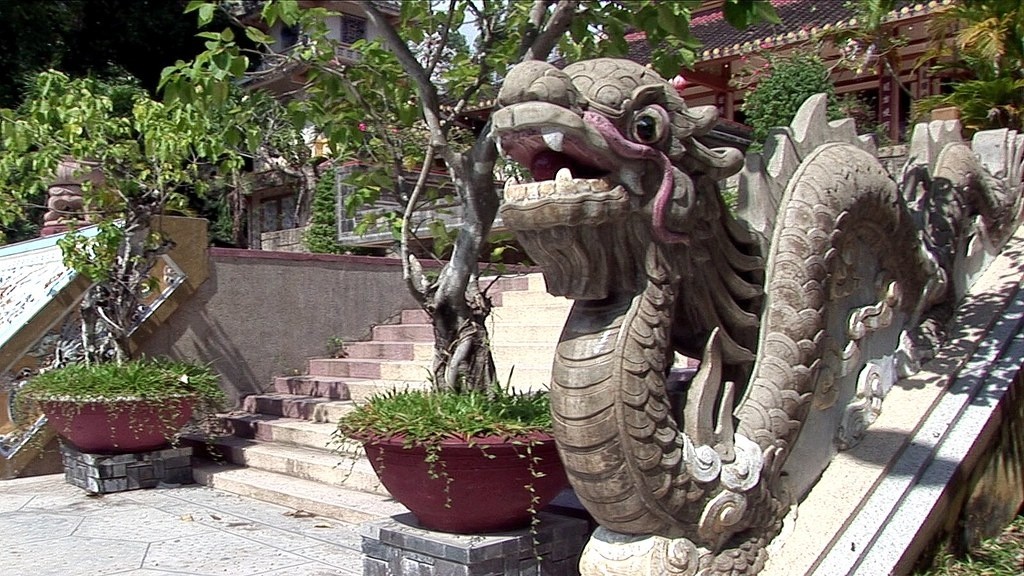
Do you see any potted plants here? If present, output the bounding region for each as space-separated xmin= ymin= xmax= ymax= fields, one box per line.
xmin=184 ymin=0 xmax=784 ymax=576
xmin=0 ymin=63 xmax=295 ymax=464
